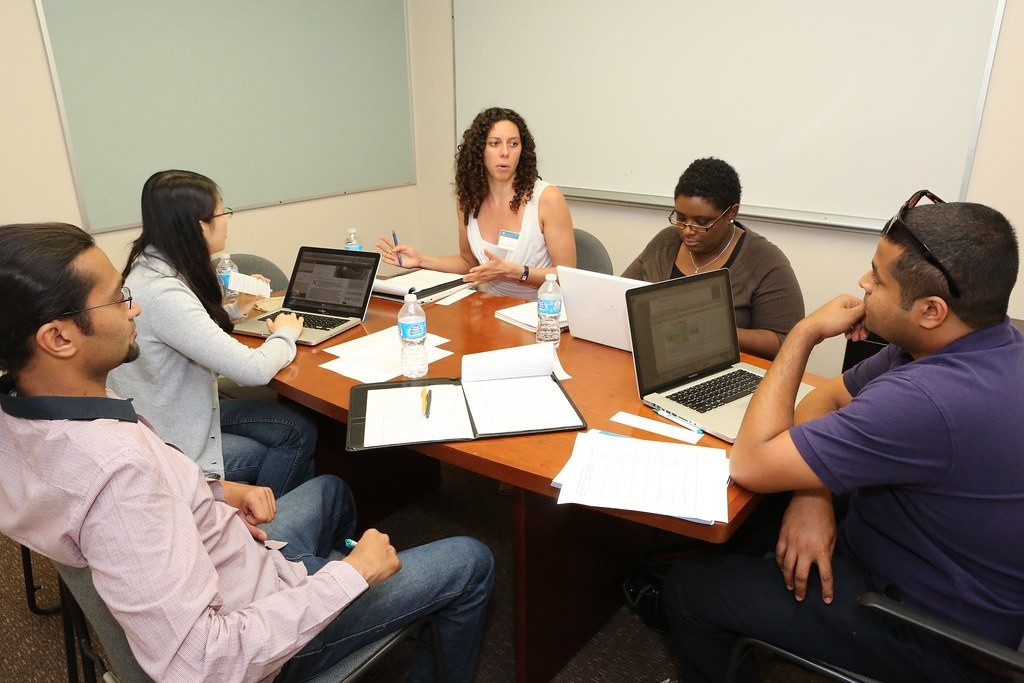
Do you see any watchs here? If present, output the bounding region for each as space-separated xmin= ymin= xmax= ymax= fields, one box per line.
xmin=517 ymin=266 xmax=529 ymax=283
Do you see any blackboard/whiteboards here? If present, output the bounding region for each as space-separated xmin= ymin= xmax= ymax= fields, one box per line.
xmin=449 ymin=0 xmax=1006 ymax=236
xmin=34 ymin=0 xmax=419 ymax=235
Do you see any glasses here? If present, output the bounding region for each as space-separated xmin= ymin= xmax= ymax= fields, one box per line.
xmin=887 ymin=189 xmax=962 ymax=298
xmin=204 ymin=206 xmax=233 ymax=222
xmin=669 ymin=205 xmax=735 ymax=234
xmin=52 ymin=287 xmax=133 ymax=316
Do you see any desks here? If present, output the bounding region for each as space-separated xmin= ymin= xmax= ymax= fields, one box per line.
xmin=196 ymin=269 xmax=853 ymax=683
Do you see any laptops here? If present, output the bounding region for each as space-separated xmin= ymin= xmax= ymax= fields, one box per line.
xmin=625 ymin=268 xmax=817 ymax=444
xmin=557 ymin=265 xmax=654 ymax=353
xmin=232 ymin=246 xmax=382 ymax=345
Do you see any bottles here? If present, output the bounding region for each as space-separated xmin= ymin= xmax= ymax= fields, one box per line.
xmin=536 ymin=273 xmax=563 ymax=349
xmin=397 ymin=294 xmax=428 ymax=378
xmin=216 ymin=253 xmax=239 ymax=321
xmin=344 ymin=229 xmax=363 ymax=251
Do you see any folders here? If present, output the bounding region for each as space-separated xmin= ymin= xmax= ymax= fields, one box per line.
xmin=346 ymin=372 xmax=588 ymax=451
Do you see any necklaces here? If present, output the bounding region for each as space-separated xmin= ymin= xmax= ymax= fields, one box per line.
xmin=689 ymin=225 xmax=735 ymax=273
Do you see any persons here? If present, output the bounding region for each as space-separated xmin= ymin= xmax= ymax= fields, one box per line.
xmin=620 ymin=189 xmax=1024 ymax=683
xmin=376 ymin=108 xmax=577 ymax=300
xmin=0 ymin=223 xmax=495 ymax=683
xmin=105 ymin=170 xmax=317 ymax=500
xmin=620 ymin=157 xmax=805 ymax=361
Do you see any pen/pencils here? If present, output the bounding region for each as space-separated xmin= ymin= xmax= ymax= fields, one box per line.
xmin=426 ymin=389 xmax=432 ymax=419
xmin=345 ymin=538 xmax=357 ymax=548
xmin=651 ymin=408 xmax=702 ymax=434
xmin=391 ymin=231 xmax=402 ymax=267
xmin=422 ymin=387 xmax=426 ymax=416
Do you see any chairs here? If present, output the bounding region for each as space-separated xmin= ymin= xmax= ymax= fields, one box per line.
xmin=572 ymin=226 xmax=614 ymax=277
xmin=729 ymin=591 xmax=1024 ymax=683
xmin=49 ymin=559 xmax=442 ymax=683
xmin=215 ymin=253 xmax=290 ymax=291
